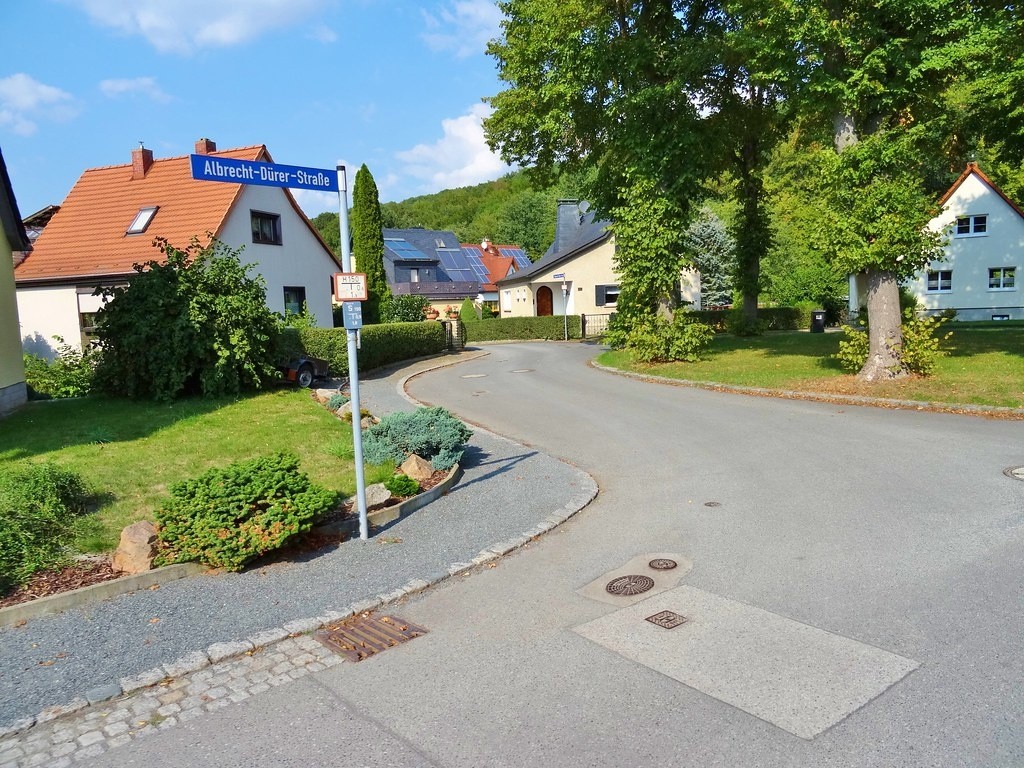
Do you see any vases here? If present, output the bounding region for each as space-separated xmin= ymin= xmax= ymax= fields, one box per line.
xmin=449 ymin=313 xmax=458 ymax=319
xmin=426 ymin=313 xmax=436 ymax=320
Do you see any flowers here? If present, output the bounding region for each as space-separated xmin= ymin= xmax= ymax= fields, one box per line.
xmin=422 ymin=306 xmax=439 ymax=317
xmin=443 ymin=305 xmax=458 ymax=318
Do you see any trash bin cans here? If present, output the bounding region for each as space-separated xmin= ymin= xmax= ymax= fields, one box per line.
xmin=810 ymin=310 xmax=826 ymax=333
